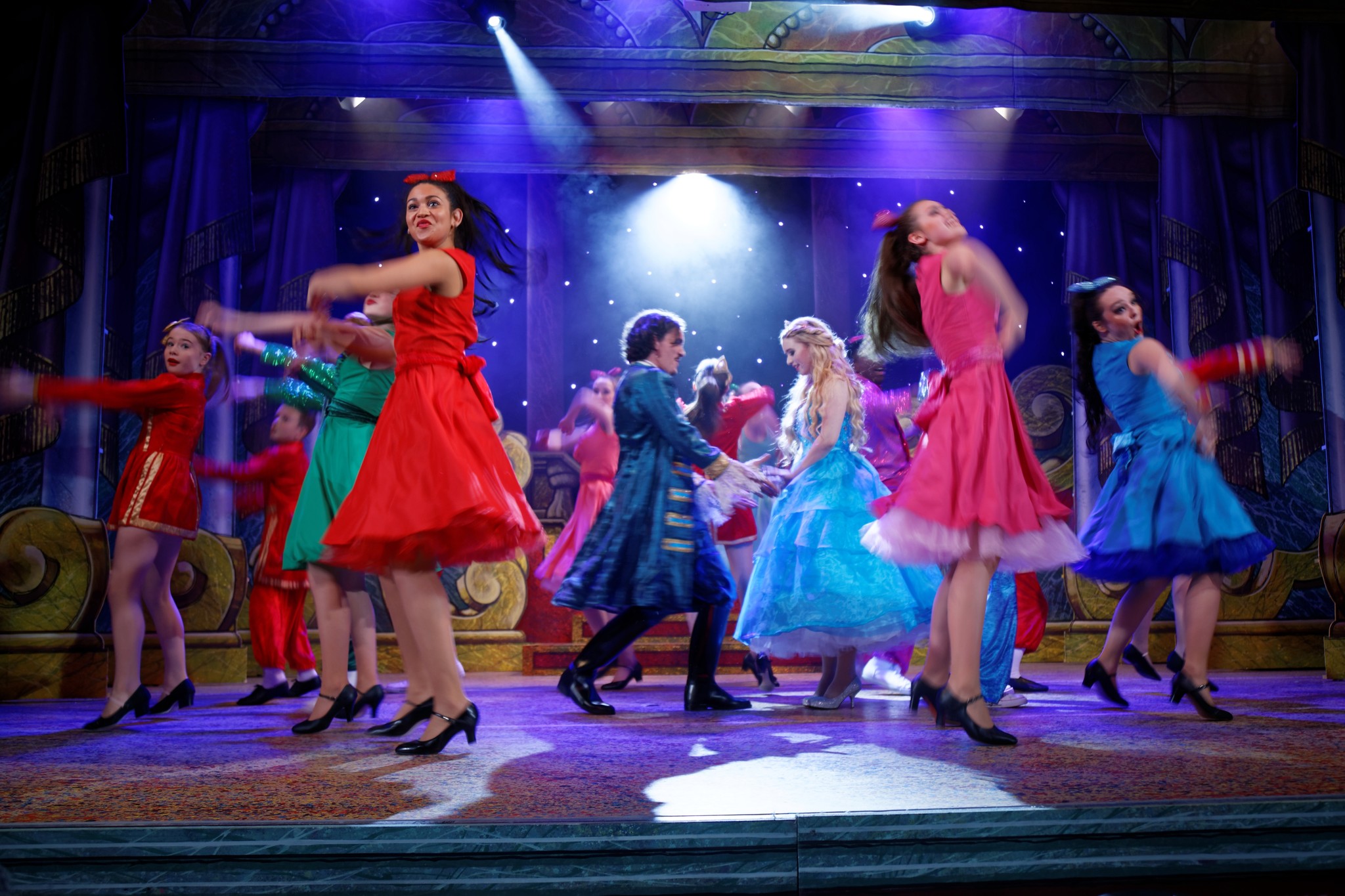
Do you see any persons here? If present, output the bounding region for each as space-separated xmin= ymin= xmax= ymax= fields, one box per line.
xmin=2 ymin=316 xmax=232 ymax=731
xmin=1065 ymin=271 xmax=1271 ymax=720
xmin=532 ymin=368 xmax=641 ymax=692
xmin=198 ymin=287 xmax=397 ymax=732
xmin=192 ymin=402 xmax=320 ymax=709
xmin=673 ymin=352 xmax=779 ymax=694
xmin=911 ymin=367 xmax=1027 ymax=711
xmin=731 ymin=317 xmax=943 ymax=712
xmin=310 ymin=167 xmax=550 ymax=757
xmin=553 ymin=300 xmax=775 ymax=713
xmin=860 ymin=200 xmax=1087 ymax=749
xmin=1122 ymin=336 xmax=1301 ymax=684
xmin=735 ymin=378 xmax=785 ymax=549
xmin=841 ymin=332 xmax=925 ymax=695
xmin=1008 ymin=565 xmax=1053 ymax=689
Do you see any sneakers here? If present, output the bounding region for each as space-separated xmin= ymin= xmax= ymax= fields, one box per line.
xmin=983 ymin=691 xmax=1028 ymax=708
xmin=861 ymin=655 xmax=914 ymax=695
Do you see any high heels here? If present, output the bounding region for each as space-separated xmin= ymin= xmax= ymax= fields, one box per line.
xmin=1168 ymin=670 xmax=1234 ymax=722
xmin=394 ymin=702 xmax=481 ymax=755
xmin=908 ymin=673 xmax=942 ymax=713
xmin=1081 ymin=656 xmax=1129 ymax=707
xmin=935 ymin=685 xmax=1018 ymax=745
xmin=81 ymin=681 xmax=153 ymax=732
xmin=148 ymin=675 xmax=196 ymax=715
xmin=739 ymin=650 xmax=781 ymax=692
xmin=802 ymin=674 xmax=863 ymax=711
xmin=593 ymin=658 xmax=642 ymax=690
xmin=290 ymin=682 xmax=358 ymax=734
xmin=335 ymin=683 xmax=385 ymax=719
xmin=364 ymin=696 xmax=434 ymax=737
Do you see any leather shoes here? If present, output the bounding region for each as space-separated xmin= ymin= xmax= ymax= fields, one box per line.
xmin=286 ymin=675 xmax=321 ymax=697
xmin=1126 ymin=642 xmax=1161 ymax=681
xmin=683 ymin=680 xmax=752 ymax=712
xmin=1166 ymin=650 xmax=1219 ymax=694
xmin=557 ymin=668 xmax=617 ymax=716
xmin=236 ymin=680 xmax=288 ymax=707
xmin=1006 ymin=674 xmax=1050 ymax=694
